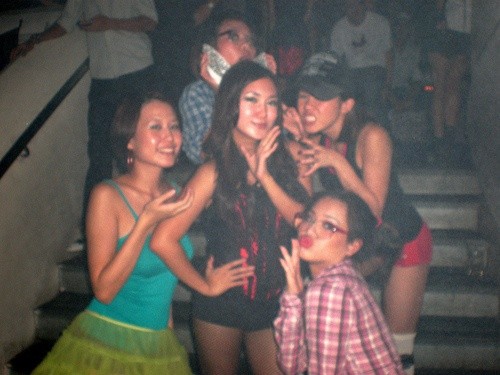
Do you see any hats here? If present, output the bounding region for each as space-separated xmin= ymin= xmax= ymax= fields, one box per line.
xmin=292 ymin=51 xmax=356 ymax=102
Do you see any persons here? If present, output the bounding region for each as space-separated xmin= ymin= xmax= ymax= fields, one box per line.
xmin=222 ymin=0 xmax=475 ymax=168
xmin=281 ymin=51 xmax=432 ymax=375
xmin=155 ymin=0 xmax=221 ymax=103
xmin=29 ymin=91 xmax=195 ymax=375
xmin=272 ymin=188 xmax=409 ymax=375
xmin=149 ymin=61 xmax=315 ymax=375
xmin=16 ymin=0 xmax=159 ymax=253
xmin=177 ymin=10 xmax=278 ymax=166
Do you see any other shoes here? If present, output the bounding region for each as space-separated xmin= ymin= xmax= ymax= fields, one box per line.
xmin=65 ymin=227 xmax=85 ymax=252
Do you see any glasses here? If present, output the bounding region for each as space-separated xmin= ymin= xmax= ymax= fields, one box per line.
xmin=217 ymin=30 xmax=255 ymax=47
xmin=292 ymin=213 xmax=351 ymax=240
